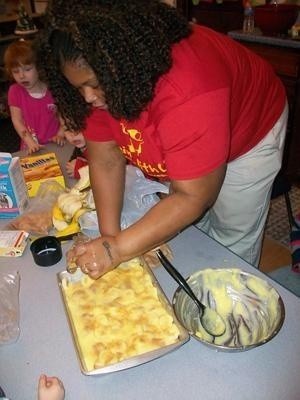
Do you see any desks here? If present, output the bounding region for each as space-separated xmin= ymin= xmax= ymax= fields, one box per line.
xmin=0 ymin=140 xmax=300 ymax=400
xmin=227 ymin=28 xmax=300 ymax=188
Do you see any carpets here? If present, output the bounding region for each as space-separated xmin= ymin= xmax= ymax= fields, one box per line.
xmin=267 ymin=264 xmax=300 ymax=296
xmin=265 ymin=185 xmax=300 ymax=249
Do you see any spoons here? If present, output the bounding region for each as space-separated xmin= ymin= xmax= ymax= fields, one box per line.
xmin=155 ymin=248 xmax=226 ymax=336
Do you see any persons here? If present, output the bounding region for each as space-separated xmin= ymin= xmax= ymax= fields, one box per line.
xmin=3 ymin=39 xmax=67 ymax=155
xmin=55 ymin=105 xmax=91 ymax=179
xmin=31 ymin=0 xmax=290 ymax=281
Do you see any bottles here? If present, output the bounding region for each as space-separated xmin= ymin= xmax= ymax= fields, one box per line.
xmin=243 ymin=7 xmax=255 ymax=32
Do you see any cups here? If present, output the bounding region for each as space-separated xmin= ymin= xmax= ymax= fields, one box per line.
xmin=29 ymin=235 xmax=63 ymax=267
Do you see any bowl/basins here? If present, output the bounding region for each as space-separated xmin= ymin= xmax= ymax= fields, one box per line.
xmin=171 ymin=269 xmax=285 ymax=352
